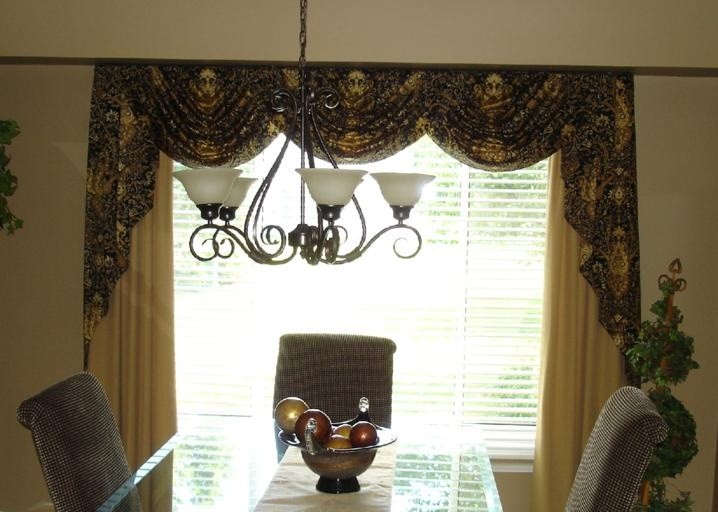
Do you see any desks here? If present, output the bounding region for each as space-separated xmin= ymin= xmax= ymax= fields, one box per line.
xmin=94 ymin=424 xmax=502 ymax=512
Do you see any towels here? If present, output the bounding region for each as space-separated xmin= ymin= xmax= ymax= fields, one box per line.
xmin=252 ymin=429 xmax=398 ymax=512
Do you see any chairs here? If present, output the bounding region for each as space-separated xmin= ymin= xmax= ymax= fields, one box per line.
xmin=16 ymin=372 xmax=142 ymax=512
xmin=563 ymin=386 xmax=670 ymax=512
xmin=273 ymin=334 xmax=396 ymax=430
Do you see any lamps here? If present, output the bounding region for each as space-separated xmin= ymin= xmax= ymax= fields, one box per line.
xmin=171 ymin=0 xmax=437 ymax=265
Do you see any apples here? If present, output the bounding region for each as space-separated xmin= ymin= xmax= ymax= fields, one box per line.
xmin=274 ymin=397 xmax=378 ymax=451
